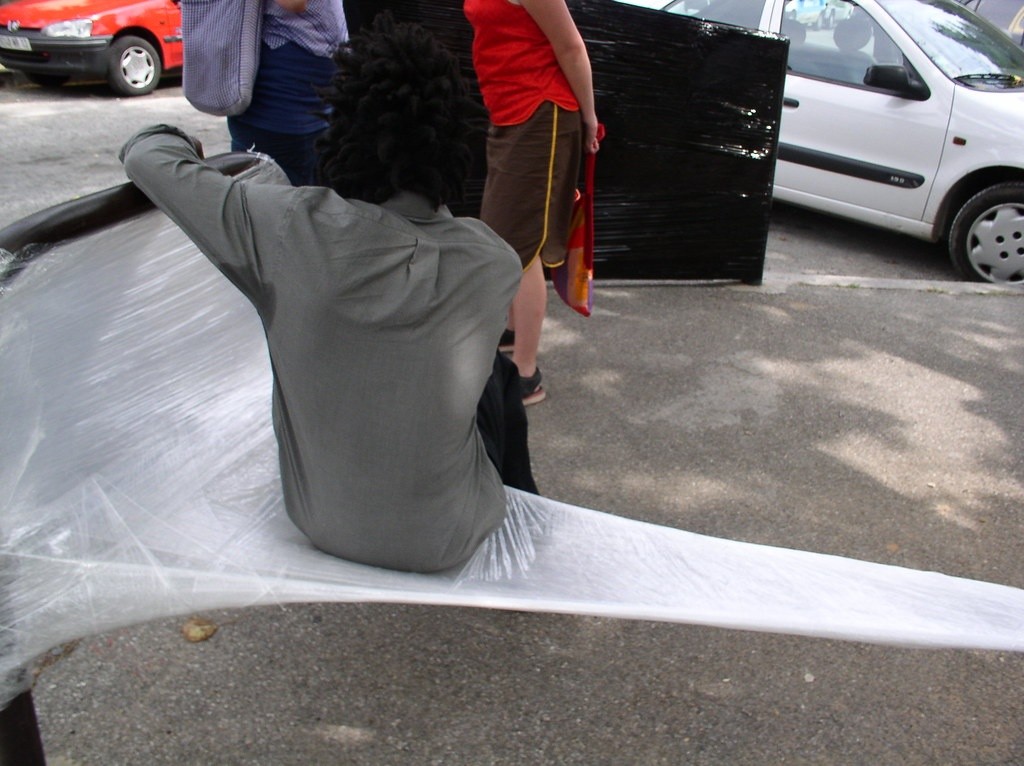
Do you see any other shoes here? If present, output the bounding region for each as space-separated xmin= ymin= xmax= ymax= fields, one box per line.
xmin=520 ymin=366 xmax=545 ymax=406
xmin=499 ymin=329 xmax=516 ymax=351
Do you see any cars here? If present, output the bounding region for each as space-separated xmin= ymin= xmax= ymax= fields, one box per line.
xmin=613 ymin=0 xmax=1024 ymax=285
xmin=0 ymin=1 xmax=186 ymax=97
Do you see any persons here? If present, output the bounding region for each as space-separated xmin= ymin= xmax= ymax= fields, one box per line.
xmin=463 ymin=0 xmax=599 ymax=407
xmin=117 ymin=8 xmax=539 ymax=573
xmin=180 ymin=0 xmax=350 ymax=187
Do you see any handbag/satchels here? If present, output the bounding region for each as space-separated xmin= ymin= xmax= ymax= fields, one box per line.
xmin=550 ymin=122 xmax=605 ymax=319
xmin=181 ymin=0 xmax=262 ymax=116
